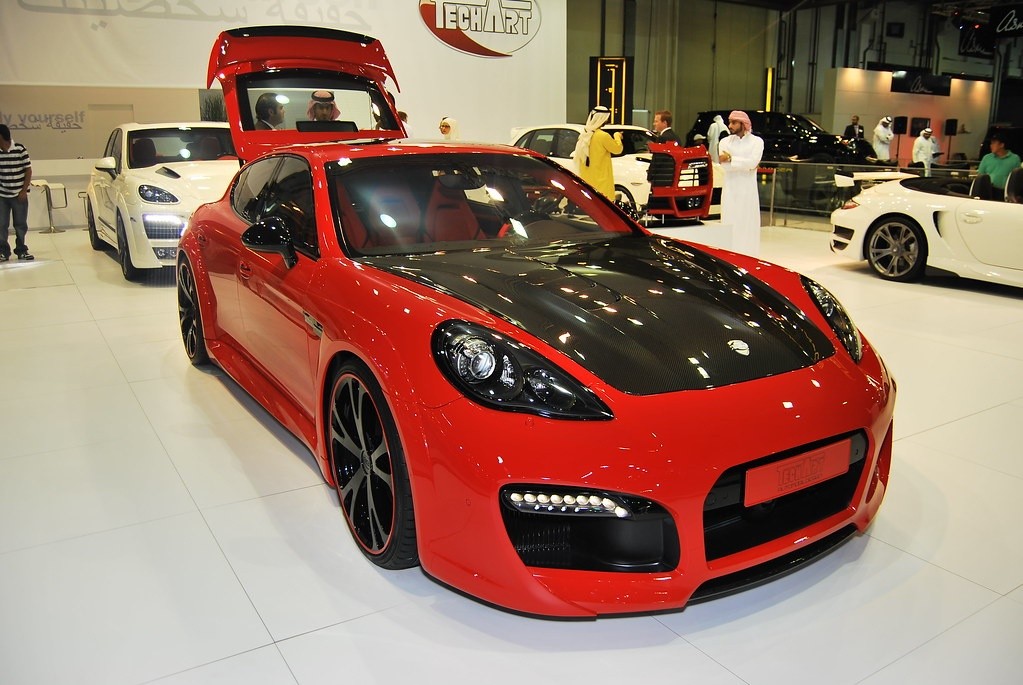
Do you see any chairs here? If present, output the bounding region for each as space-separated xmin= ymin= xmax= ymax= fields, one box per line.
xmin=370 ymin=180 xmax=425 ymax=251
xmin=132 ymin=139 xmax=157 ymax=167
xmin=1003 ymin=168 xmax=1023 ymax=204
xmin=970 ymin=173 xmax=996 ymax=199
xmin=196 ymin=138 xmax=223 ymax=159
xmin=300 ymin=182 xmax=366 ymax=252
xmin=420 ymin=175 xmax=486 ymax=243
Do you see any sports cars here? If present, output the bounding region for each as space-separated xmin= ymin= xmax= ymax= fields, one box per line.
xmin=829 ymin=162 xmax=1023 ymax=294
xmin=176 ymin=25 xmax=897 ymax=620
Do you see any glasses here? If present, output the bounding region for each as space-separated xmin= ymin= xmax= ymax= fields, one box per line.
xmin=440 ymin=125 xmax=450 ymax=129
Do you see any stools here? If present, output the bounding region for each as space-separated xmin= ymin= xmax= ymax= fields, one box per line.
xmin=30 ymin=180 xmax=68 ymax=233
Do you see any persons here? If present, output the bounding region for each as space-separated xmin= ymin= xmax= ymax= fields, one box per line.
xmin=306 ymin=91 xmax=341 ymax=121
xmin=694 ymin=134 xmax=706 ymax=146
xmin=844 ymin=116 xmax=864 ymax=138
xmin=255 ymin=93 xmax=285 ymax=129
xmin=719 ymin=111 xmax=764 ymax=258
xmin=371 ymin=91 xmax=414 ymax=138
xmin=977 ymin=133 xmax=1021 ymax=189
xmin=0 ymin=123 xmax=32 ymax=262
xmin=574 ymin=106 xmax=623 ymax=202
xmin=707 ymin=114 xmax=731 ymax=162
xmin=912 ymin=128 xmax=940 ymax=177
xmin=438 ymin=117 xmax=459 ymax=140
xmin=647 ymin=110 xmax=681 ymax=187
xmin=873 ymin=116 xmax=894 ymax=159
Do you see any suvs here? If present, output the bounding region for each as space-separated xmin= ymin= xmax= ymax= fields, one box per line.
xmin=685 ymin=110 xmax=877 ymax=195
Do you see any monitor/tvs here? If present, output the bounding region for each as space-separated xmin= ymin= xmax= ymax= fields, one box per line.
xmin=910 ymin=118 xmax=930 ymax=137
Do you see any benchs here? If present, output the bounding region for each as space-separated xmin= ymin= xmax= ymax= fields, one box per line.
xmin=158 ymin=154 xmax=182 ymax=163
xmin=925 ymin=178 xmax=970 ymax=196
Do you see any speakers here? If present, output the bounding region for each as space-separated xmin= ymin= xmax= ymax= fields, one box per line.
xmin=893 ymin=116 xmax=907 ymax=135
xmin=945 ymin=119 xmax=957 ymax=136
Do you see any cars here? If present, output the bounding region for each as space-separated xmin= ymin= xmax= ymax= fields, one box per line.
xmin=507 ymin=124 xmax=697 ymax=225
xmin=85 ymin=121 xmax=293 ymax=281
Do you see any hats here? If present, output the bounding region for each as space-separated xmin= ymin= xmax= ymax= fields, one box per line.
xmin=990 ymin=132 xmax=1008 ymax=146
xmin=728 ymin=111 xmax=752 ymax=133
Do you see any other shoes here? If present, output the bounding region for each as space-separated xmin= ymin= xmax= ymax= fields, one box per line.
xmin=18 ymin=251 xmax=34 ymax=260
xmin=0 ymin=253 xmax=10 ymax=262
